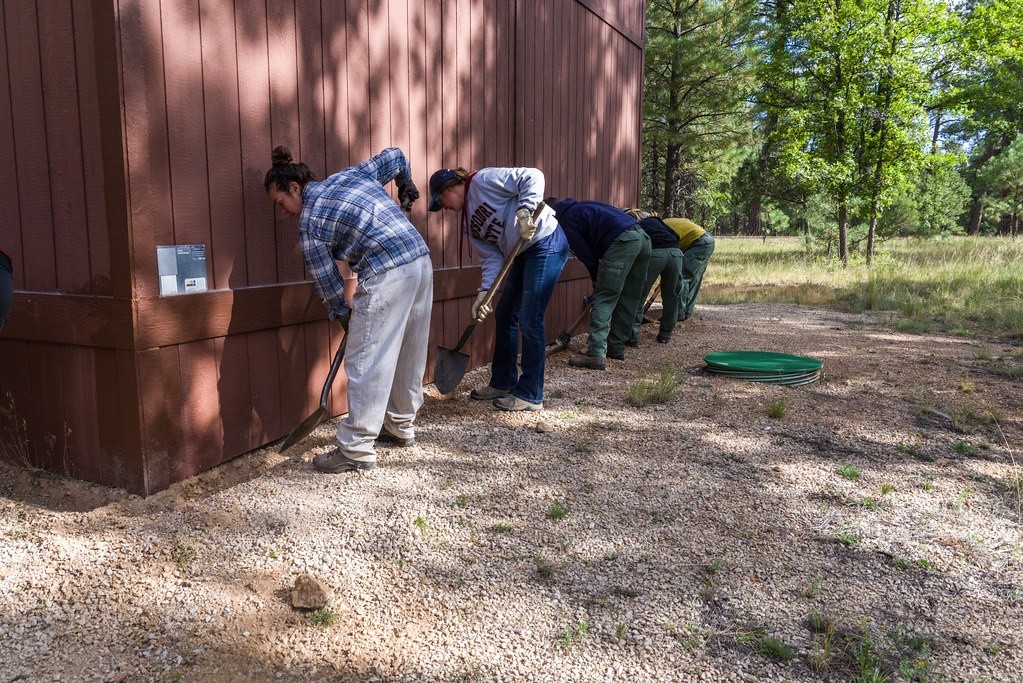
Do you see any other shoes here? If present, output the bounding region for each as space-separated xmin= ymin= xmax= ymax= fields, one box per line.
xmin=658 ymin=313 xmax=685 ymax=321
xmin=657 ymin=335 xmax=670 ymax=343
xmin=624 ymin=341 xmax=638 ymax=347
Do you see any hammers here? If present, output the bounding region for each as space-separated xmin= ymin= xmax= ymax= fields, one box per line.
xmin=642 ymin=284 xmax=663 ymax=323
xmin=555 ymin=301 xmax=594 ymax=348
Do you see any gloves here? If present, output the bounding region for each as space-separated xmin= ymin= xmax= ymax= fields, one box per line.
xmin=339 ymin=308 xmax=352 ymax=333
xmin=398 ymin=179 xmax=419 ymax=212
xmin=515 ymin=208 xmax=539 ymax=241
xmin=472 ymin=292 xmax=494 ymax=323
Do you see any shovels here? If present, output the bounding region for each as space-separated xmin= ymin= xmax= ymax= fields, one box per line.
xmin=435 ymin=199 xmax=547 ymax=395
xmin=275 ymin=193 xmax=413 ymax=453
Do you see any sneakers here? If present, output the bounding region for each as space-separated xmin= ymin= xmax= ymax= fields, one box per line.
xmin=377 ymin=424 xmax=415 ymax=447
xmin=606 ymin=352 xmax=624 ymax=361
xmin=312 ymin=446 xmax=375 ymax=473
xmin=472 ymin=385 xmax=512 ymax=399
xmin=492 ymin=395 xmax=544 ymax=411
xmin=568 ymin=355 xmax=606 ymax=369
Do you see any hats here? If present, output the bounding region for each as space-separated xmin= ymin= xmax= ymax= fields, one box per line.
xmin=426 ymin=169 xmax=456 ymax=212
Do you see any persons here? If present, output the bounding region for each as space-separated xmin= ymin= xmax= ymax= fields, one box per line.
xmin=543 ymin=198 xmax=652 ymax=370
xmin=428 ymin=168 xmax=569 ymax=410
xmin=622 ymin=207 xmax=715 ymax=348
xmin=265 ymin=145 xmax=433 ymax=472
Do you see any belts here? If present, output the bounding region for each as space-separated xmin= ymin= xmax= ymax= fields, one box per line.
xmin=627 ymin=224 xmax=642 ymax=230
xmin=691 ymin=233 xmax=706 ymax=244
